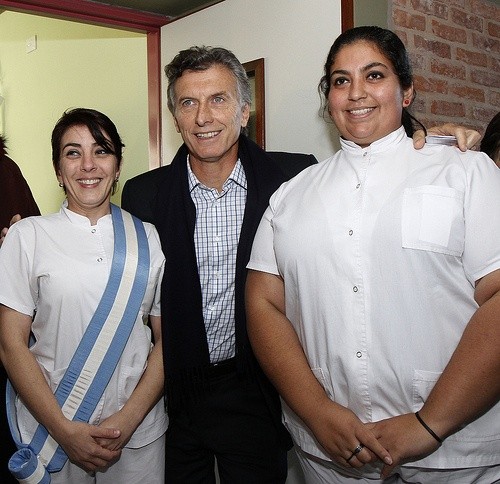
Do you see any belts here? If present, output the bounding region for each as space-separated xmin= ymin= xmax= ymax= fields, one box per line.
xmin=210 ymin=357 xmax=234 ymax=379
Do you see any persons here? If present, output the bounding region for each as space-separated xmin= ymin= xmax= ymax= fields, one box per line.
xmin=0 ymin=107 xmax=169 ymax=483
xmin=244 ymin=25 xmax=500 ymax=484
xmin=2 ymin=47 xmax=482 ymax=484
xmin=0 ymin=134 xmax=42 ymax=483
xmin=478 ymin=106 xmax=500 ymax=168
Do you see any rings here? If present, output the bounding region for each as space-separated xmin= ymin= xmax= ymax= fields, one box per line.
xmin=353 ymin=443 xmax=364 ymax=453
xmin=345 ymin=453 xmax=353 ymax=462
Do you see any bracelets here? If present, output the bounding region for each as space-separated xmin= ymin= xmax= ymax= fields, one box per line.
xmin=414 ymin=412 xmax=443 ymax=445
